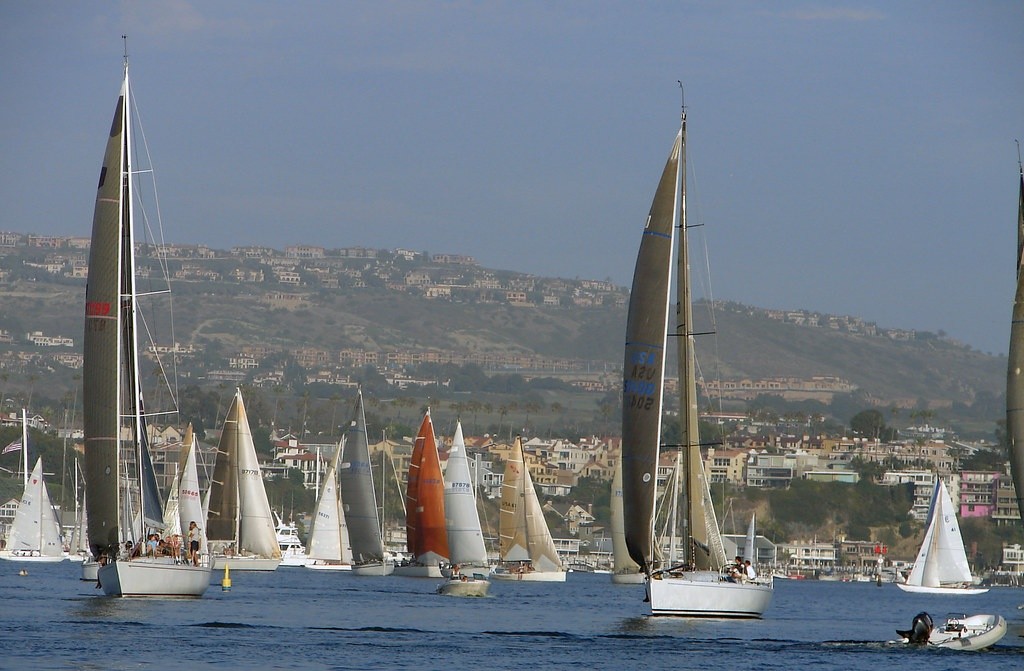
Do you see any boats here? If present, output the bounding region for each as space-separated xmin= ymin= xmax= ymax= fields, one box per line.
xmin=270 ymin=492 xmax=305 ymax=567
xmin=895 ymin=611 xmax=1008 ymax=652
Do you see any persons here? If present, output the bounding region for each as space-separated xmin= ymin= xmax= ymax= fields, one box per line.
xmin=728 ymin=556 xmax=747 ymax=579
xmin=95 ymin=521 xmax=199 ymax=592
xmin=746 ymin=560 xmax=757 ymax=579
xmin=489 ymin=561 xmax=532 ymax=572
xmin=394 ymin=554 xmax=416 ymax=566
xmin=23 ymin=553 xmax=25 ymax=556
xmin=439 ymin=560 xmax=468 ymax=581
xmin=314 ymin=560 xmax=317 ymax=564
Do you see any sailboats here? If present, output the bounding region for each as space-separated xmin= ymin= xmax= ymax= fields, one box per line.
xmin=340 ymin=384 xmax=395 ymax=576
xmin=394 ymin=406 xmax=451 ymax=577
xmin=438 ymin=420 xmax=488 ymax=597
xmin=486 ymin=436 xmax=568 ymax=583
xmin=204 ymin=388 xmax=282 ymax=572
xmin=303 ymin=434 xmax=351 ymax=570
xmin=895 ymin=470 xmax=990 ymax=594
xmin=617 ymin=80 xmax=777 ymax=618
xmin=0 ymin=31 xmax=213 ymax=597
xmin=608 ymin=439 xmax=646 ymax=585
